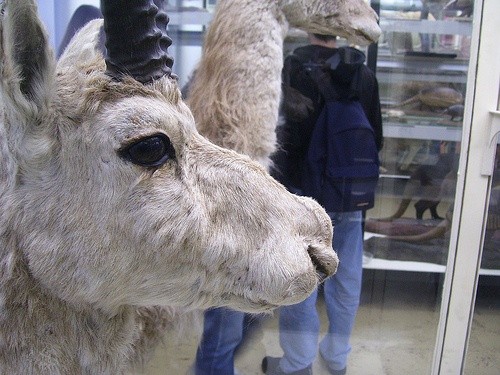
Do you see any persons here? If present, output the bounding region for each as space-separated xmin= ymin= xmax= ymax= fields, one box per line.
xmin=258 ymin=32 xmax=383 ymax=375
xmin=194 ymin=304 xmax=254 ymax=375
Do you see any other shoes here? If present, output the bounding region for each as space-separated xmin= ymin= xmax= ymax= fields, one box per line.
xmin=319 ymin=348 xmax=346 ymax=375
xmin=262 ymin=356 xmax=312 ymax=375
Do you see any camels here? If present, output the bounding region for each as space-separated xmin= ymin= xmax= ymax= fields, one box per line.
xmin=1 ymin=0 xmax=340 ymax=373
xmin=182 ymin=0 xmax=382 ymax=177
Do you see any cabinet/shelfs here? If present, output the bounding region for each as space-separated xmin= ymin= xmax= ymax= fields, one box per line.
xmin=366 ymin=0 xmax=500 ymax=277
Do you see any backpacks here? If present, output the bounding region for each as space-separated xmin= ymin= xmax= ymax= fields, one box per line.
xmin=288 ymin=50 xmax=379 ymax=212
